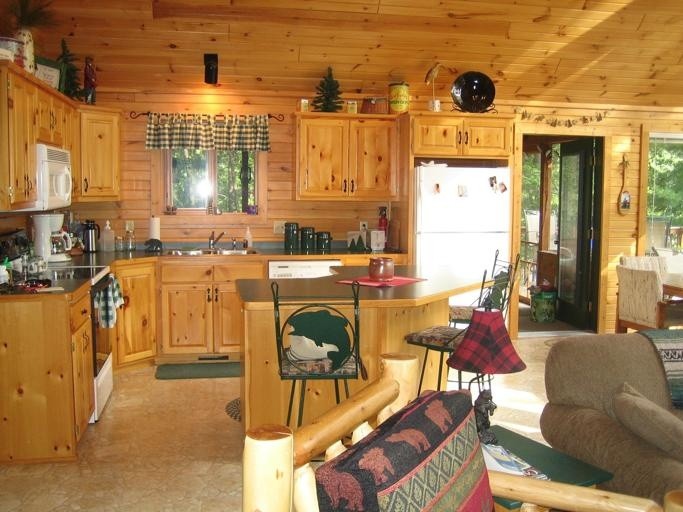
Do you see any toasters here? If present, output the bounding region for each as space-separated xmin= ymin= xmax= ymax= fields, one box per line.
xmin=346 ymin=230 xmax=385 ymax=253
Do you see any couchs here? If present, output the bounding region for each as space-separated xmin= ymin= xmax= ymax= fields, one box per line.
xmin=528 ymin=318 xmax=682 ymax=511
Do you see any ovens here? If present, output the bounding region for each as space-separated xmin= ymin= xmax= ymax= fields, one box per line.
xmin=90 ymin=277 xmax=114 ymax=424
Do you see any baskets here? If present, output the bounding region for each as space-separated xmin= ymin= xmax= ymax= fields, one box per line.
xmin=360 ymin=97 xmax=388 ymax=114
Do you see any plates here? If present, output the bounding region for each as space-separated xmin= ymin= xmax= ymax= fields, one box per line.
xmin=451 ymin=70 xmax=495 ymax=110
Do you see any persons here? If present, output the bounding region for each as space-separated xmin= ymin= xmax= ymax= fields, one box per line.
xmin=84 ymin=55 xmax=96 ymax=105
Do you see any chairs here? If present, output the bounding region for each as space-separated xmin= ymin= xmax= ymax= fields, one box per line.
xmin=403 ymin=262 xmax=513 ymax=402
xmin=444 ymin=250 xmax=521 ymax=335
xmin=232 ymin=367 xmax=662 ymax=512
xmin=618 ymin=255 xmax=674 ymax=303
xmin=269 ymin=281 xmax=368 ymax=426
xmin=614 ymin=265 xmax=682 ymax=339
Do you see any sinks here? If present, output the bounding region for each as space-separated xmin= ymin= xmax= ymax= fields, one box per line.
xmin=165 ymin=248 xmax=259 ymax=255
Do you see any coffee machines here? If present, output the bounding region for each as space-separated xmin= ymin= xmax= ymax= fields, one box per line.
xmin=30 ymin=214 xmax=75 ymax=262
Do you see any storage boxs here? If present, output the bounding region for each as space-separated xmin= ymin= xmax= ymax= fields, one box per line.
xmin=88 ymin=350 xmax=114 ymax=426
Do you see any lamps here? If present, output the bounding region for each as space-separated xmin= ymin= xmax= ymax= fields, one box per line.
xmin=613 ymin=134 xmax=634 ymax=188
xmin=442 ymin=288 xmax=530 ymax=433
xmin=201 ymin=52 xmax=219 ymax=86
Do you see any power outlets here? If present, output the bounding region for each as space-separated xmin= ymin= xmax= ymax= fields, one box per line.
xmin=359 ymin=221 xmax=368 ymax=231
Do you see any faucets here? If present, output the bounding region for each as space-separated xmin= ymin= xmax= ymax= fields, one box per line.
xmin=208 ymin=231 xmax=224 ymax=248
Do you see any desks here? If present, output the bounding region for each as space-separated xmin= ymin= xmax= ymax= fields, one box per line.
xmin=475 ymin=422 xmax=616 ymax=511
xmin=229 ymin=261 xmax=501 ymax=442
xmin=659 ymin=270 xmax=682 ymax=301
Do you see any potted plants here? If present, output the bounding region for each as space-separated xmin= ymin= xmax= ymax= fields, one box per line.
xmin=5 ymin=0 xmax=58 ymax=76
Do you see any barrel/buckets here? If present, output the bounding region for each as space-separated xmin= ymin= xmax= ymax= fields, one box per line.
xmin=530 ymin=289 xmax=557 ymax=324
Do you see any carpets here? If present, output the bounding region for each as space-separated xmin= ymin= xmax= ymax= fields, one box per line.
xmin=223 ymin=397 xmax=241 ymax=424
xmin=152 ymin=360 xmax=242 ymax=381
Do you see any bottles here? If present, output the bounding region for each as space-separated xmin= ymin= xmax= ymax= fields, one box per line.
xmin=283 ymin=222 xmax=300 ymax=250
xmin=115 ymin=236 xmax=123 ymax=252
xmin=101 ymin=221 xmax=114 ymax=253
xmin=314 ymin=232 xmax=332 ymax=251
xmin=21 ymin=252 xmax=29 ymax=274
xmin=2 ymin=256 xmax=13 ymax=282
xmin=207 ymin=198 xmax=213 ymax=215
xmin=300 ymin=227 xmax=314 ymax=251
xmin=124 ymin=230 xmax=135 ymax=250
xmin=378 ymin=207 xmax=388 ymax=245
xmin=344 ymin=101 xmax=358 ymax=114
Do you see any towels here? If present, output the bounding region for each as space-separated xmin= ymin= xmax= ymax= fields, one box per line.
xmin=94 ymin=274 xmax=126 ymax=332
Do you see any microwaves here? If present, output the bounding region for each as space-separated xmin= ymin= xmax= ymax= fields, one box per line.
xmin=11 ymin=144 xmax=72 ymax=212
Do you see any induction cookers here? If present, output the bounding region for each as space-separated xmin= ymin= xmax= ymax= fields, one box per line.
xmin=30 ymin=264 xmax=110 ymax=287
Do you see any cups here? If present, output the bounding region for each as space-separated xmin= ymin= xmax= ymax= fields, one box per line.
xmin=369 ymin=257 xmax=394 ymax=282
xmin=171 ymin=207 xmax=176 ymax=211
xmin=665 ymin=486 xmax=683 ymax=511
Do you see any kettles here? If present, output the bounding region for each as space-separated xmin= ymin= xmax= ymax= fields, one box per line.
xmin=80 ymin=219 xmax=100 ymax=254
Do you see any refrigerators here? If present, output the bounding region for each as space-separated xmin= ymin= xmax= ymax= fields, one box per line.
xmin=413 ymin=165 xmax=513 ymax=335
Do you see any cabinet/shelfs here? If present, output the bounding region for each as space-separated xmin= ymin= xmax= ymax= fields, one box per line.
xmin=113 ymin=257 xmax=157 ymax=370
xmin=0 ymin=58 xmax=37 ymax=214
xmin=62 ymin=98 xmax=81 ymax=205
xmin=155 ymin=253 xmax=407 ymax=356
xmin=0 ymin=280 xmax=94 ymax=468
xmin=289 ymin=111 xmax=403 ymax=203
xmin=73 ymin=99 xmax=122 ymax=205
xmin=37 ymin=76 xmax=67 ymax=148
xmin=404 ymin=108 xmax=516 ymax=159
xmin=535 ymin=248 xmax=556 ymax=292
